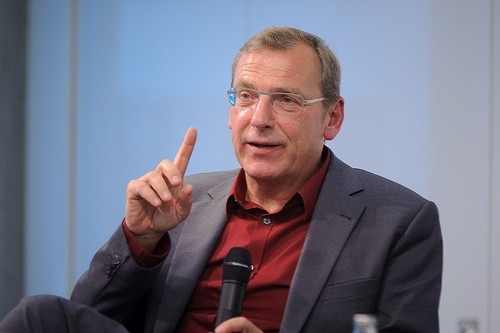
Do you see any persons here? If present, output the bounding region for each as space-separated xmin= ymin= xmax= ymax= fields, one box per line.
xmin=1 ymin=26 xmax=444 ymax=333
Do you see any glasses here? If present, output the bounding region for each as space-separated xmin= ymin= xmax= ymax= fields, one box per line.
xmin=226 ymin=86 xmax=324 ymax=114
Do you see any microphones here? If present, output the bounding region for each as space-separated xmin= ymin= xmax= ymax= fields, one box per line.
xmin=215 ymin=247 xmax=252 ymax=328
